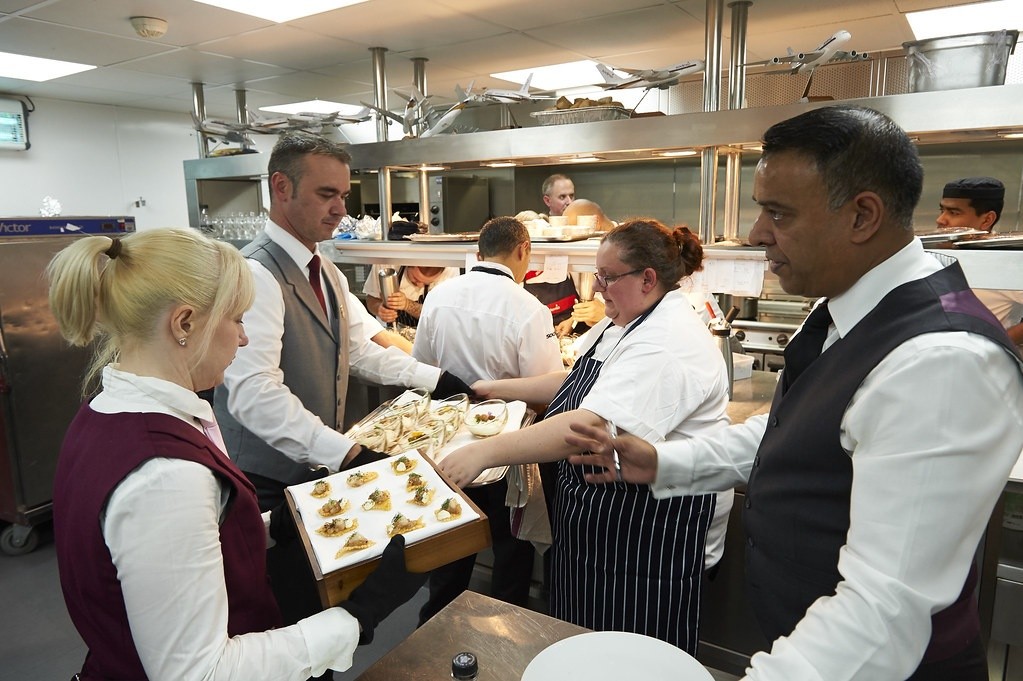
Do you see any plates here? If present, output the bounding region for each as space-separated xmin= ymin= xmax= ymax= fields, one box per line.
xmin=520 ymin=631 xmax=716 ymax=681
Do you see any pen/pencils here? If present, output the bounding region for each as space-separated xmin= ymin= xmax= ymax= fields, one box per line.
xmin=605 ymin=420 xmax=621 ymax=482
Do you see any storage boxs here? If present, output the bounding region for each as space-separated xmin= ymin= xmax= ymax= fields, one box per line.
xmin=732 ymin=352 xmax=755 ymax=381
xmin=284 ymin=448 xmax=494 ymax=611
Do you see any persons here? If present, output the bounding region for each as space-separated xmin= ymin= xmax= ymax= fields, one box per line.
xmin=523 ymin=174 xmax=591 ymax=336
xmin=213 ymin=129 xmax=487 ymax=607
xmin=934 ymin=175 xmax=1023 ymax=345
xmin=566 ymin=104 xmax=1023 ymax=681
xmin=44 ymin=228 xmax=428 ymax=681
xmin=413 ymin=219 xmax=566 ymax=632
xmin=361 ymin=264 xmax=461 ymax=326
xmin=437 ymin=217 xmax=734 ymax=659
xmin=550 ymin=199 xmax=627 ymax=336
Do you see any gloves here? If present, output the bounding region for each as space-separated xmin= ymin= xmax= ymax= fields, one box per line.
xmin=269 ymin=465 xmax=330 ymax=542
xmin=336 ymin=534 xmax=431 ymax=645
xmin=343 ymin=443 xmax=392 ymax=469
xmin=432 ymin=370 xmax=476 ymax=401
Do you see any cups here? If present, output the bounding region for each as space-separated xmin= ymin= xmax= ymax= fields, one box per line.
xmin=524 ymin=220 xmax=544 ymax=237
xmin=549 ymin=216 xmax=567 ymax=234
xmin=578 ymin=216 xmax=596 ymax=232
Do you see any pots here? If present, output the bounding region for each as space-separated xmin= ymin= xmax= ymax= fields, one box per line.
xmin=902 ymin=30 xmax=1020 ymax=93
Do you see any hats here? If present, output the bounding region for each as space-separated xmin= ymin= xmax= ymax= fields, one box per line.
xmin=943 ymin=176 xmax=1006 ymax=200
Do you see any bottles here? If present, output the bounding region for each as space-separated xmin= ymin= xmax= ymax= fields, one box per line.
xmin=450 ymin=652 xmax=479 ymax=681
xmin=201 ymin=209 xmax=269 ymax=240
xmin=580 ymin=272 xmax=595 ymax=303
xmin=379 ymin=268 xmax=399 ymax=322
xmin=705 ymin=302 xmax=740 ymax=400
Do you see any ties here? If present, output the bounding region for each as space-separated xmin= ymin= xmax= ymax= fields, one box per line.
xmin=308 ymin=254 xmax=328 ymax=322
xmin=781 ymin=299 xmax=833 ymax=392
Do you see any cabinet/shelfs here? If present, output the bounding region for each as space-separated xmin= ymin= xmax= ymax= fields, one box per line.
xmin=182 ymin=82 xmax=1023 ymax=681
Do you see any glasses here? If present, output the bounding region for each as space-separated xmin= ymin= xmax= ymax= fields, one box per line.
xmin=594 ymin=269 xmax=643 ymax=288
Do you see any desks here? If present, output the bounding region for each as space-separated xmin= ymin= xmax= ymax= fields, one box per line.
xmin=354 ymin=590 xmax=740 ymax=681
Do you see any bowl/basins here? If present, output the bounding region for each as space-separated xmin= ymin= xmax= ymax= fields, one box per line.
xmin=545 ymin=226 xmax=564 ymax=238
xmin=564 ymin=226 xmax=590 ymax=238
xmin=351 ymin=388 xmax=431 ymax=455
xmin=464 ymin=399 xmax=508 ymax=438
xmin=388 ymin=393 xmax=469 ymax=457
